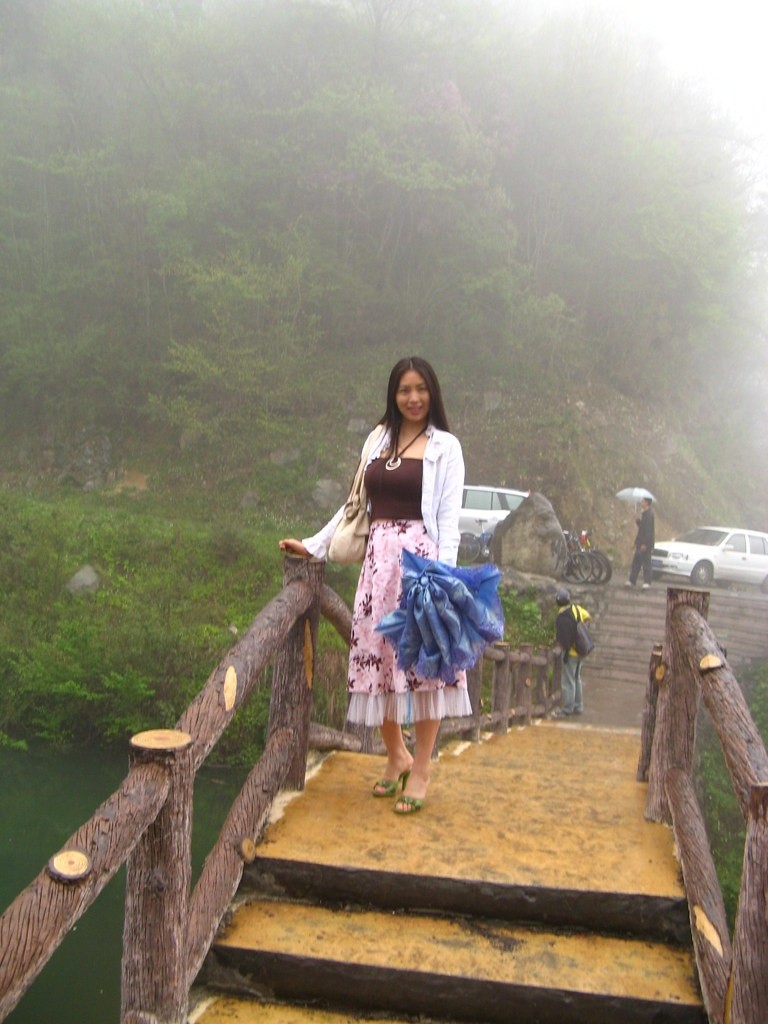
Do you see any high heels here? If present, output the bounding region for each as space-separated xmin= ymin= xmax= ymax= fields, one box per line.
xmin=372 ymin=768 xmax=412 ymax=798
xmin=393 ymin=794 xmax=423 ymax=815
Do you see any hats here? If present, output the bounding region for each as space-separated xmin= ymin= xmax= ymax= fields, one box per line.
xmin=556 ymin=588 xmax=570 ymax=604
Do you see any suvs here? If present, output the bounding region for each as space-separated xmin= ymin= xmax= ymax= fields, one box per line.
xmin=458 ymin=486 xmax=532 ymax=539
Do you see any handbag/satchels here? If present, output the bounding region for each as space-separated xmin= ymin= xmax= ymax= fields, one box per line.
xmin=574 ymin=604 xmax=594 ymax=655
xmin=329 ymin=425 xmax=382 ymax=563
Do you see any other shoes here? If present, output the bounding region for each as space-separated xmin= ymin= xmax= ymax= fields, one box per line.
xmin=553 ymin=710 xmax=568 ymax=716
xmin=625 ymin=581 xmax=632 ymax=587
xmin=574 ymin=710 xmax=582 ymax=714
xmin=643 ymin=583 xmax=649 ymax=588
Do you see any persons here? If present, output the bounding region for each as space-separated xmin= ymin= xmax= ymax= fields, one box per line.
xmin=278 ymin=357 xmax=473 ymax=814
xmin=554 ymin=590 xmax=592 ymax=716
xmin=625 ymin=497 xmax=654 ymax=589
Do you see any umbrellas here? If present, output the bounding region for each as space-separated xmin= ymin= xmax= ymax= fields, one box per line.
xmin=616 ymin=487 xmax=657 ymax=513
xmin=372 ymin=546 xmax=504 ymax=686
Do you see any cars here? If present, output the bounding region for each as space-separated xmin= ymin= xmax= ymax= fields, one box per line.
xmin=647 ymin=526 xmax=768 ymax=588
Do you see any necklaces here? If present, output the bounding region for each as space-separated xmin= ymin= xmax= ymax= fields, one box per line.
xmin=385 ymin=423 xmax=428 ymax=471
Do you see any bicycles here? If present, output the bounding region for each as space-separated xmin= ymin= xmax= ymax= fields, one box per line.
xmin=457 ymin=519 xmax=495 ymax=566
xmin=558 ymin=529 xmax=613 ymax=586
